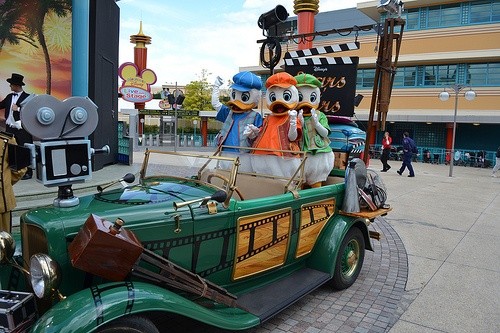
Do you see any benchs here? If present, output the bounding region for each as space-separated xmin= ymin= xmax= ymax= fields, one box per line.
xmin=199 ymin=168 xmax=294 ymax=202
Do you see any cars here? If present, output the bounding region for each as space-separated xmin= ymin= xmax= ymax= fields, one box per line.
xmin=0 ymin=140 xmax=394 ymax=333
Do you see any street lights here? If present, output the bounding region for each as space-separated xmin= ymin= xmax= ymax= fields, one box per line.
xmin=169 ymin=81 xmax=185 ymax=151
xmin=438 ymin=83 xmax=476 ymax=177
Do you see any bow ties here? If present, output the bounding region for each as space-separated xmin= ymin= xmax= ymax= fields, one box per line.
xmin=12 ymin=93 xmax=18 ymax=96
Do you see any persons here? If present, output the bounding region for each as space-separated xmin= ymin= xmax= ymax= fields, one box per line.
xmin=0 ymin=131 xmax=27 ymax=233
xmin=0 ymin=73 xmax=33 ymax=180
xmin=492 ymin=146 xmax=500 ymax=176
xmin=477 ymin=149 xmax=487 ymax=168
xmin=423 ymin=149 xmax=431 ymax=163
xmin=381 ymin=132 xmax=392 ymax=172
xmin=397 ymin=132 xmax=418 ymax=177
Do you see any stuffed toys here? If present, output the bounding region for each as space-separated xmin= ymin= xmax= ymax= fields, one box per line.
xmin=294 ymin=74 xmax=334 ymax=188
xmin=212 ymin=71 xmax=263 ymax=173
xmin=249 ymin=71 xmax=301 ymax=178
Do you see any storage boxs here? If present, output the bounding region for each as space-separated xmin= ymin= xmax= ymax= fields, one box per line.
xmin=0 ymin=289 xmax=38 ymax=333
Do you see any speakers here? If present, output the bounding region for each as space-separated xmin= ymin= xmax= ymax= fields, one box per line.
xmin=176 ymin=95 xmax=185 ymax=105
xmin=168 ymin=94 xmax=174 ymax=104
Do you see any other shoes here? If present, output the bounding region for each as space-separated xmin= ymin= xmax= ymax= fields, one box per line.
xmin=21 ymin=173 xmax=31 ymax=180
xmin=387 ymin=166 xmax=391 ymax=170
xmin=397 ymin=171 xmax=402 ymax=175
xmin=381 ymin=170 xmax=387 ymax=172
xmin=408 ymin=175 xmax=414 ymax=177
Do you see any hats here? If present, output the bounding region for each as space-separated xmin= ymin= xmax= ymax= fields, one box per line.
xmin=6 ymin=73 xmax=25 ymax=86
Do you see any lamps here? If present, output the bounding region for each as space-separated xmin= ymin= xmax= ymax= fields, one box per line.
xmin=258 ymin=5 xmax=290 ymax=37
xmin=376 ymin=0 xmax=405 ymax=19
